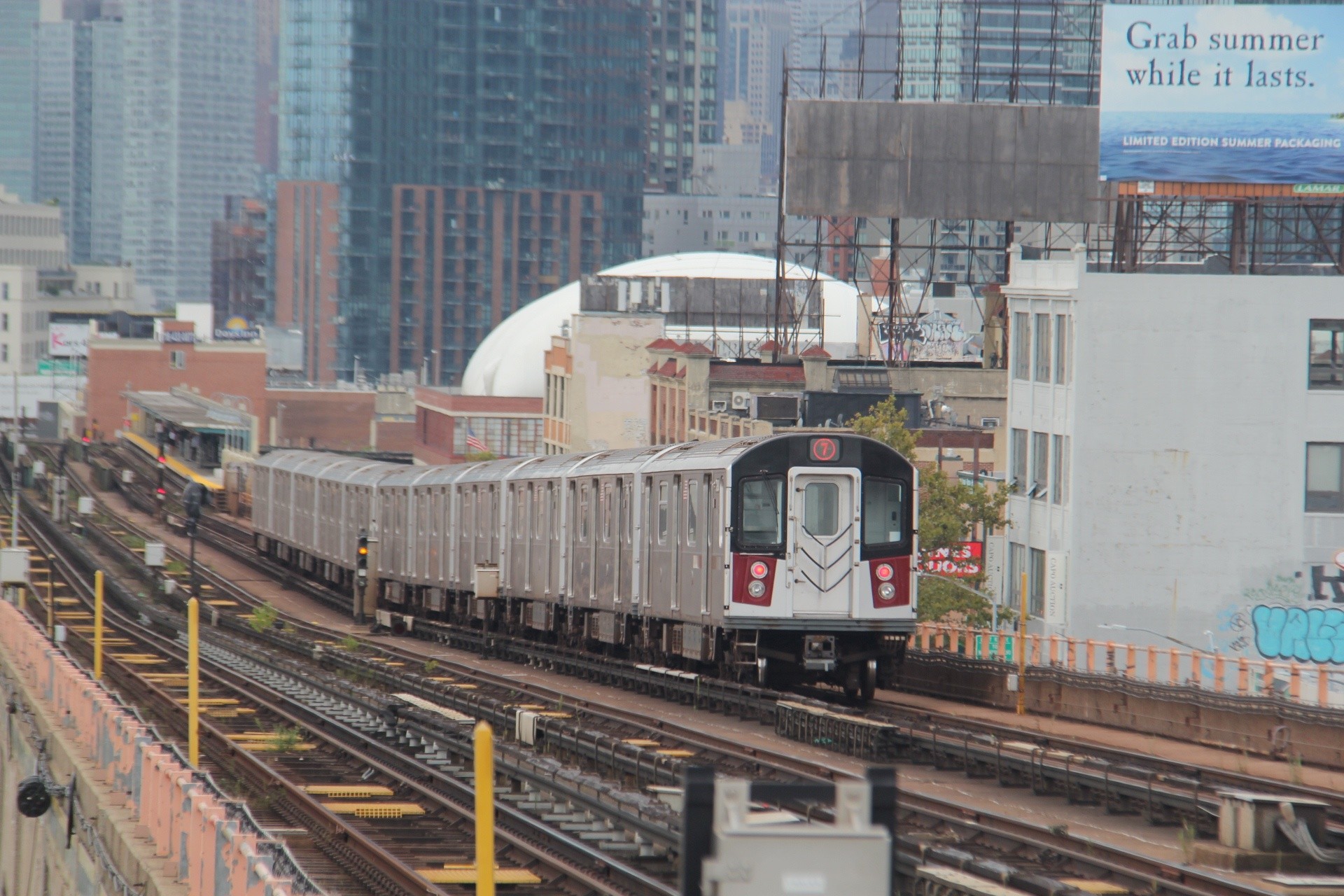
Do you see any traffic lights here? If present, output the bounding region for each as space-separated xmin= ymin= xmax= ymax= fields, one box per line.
xmin=356 ymin=535 xmax=368 ymax=570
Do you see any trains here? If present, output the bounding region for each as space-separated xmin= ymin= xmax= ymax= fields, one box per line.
xmin=118 ymin=387 xmax=261 ymax=511
xmin=254 ymin=425 xmax=921 ymax=704
